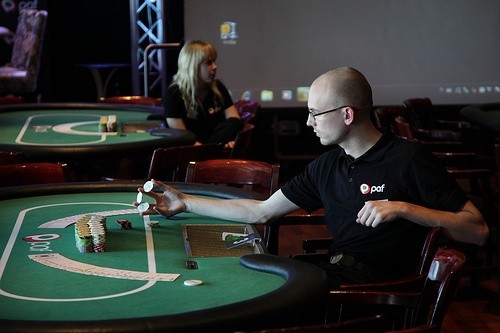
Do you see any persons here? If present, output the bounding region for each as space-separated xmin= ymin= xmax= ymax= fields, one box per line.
xmin=164 ymin=39 xmax=245 ymax=149
xmin=133 ymin=65 xmax=489 ymax=333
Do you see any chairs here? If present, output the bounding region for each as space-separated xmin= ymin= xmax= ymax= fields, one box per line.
xmin=146 ymin=98 xmax=500 ymax=333
xmin=0 ymin=7 xmax=48 ymax=103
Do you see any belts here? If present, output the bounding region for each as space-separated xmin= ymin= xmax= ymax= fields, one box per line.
xmin=329 ymin=252 xmax=367 ymax=271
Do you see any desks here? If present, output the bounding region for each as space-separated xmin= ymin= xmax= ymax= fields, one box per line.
xmin=0 ymin=179 xmax=326 ymax=333
xmin=80 ymin=65 xmax=129 ymax=103
xmin=0 ymin=102 xmax=197 ymax=188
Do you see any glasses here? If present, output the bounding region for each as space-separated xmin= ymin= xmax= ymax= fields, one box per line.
xmin=307 ymin=105 xmax=359 ymax=119
